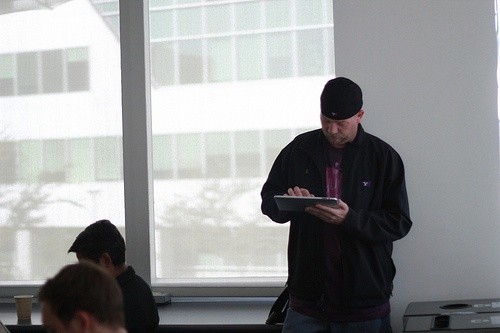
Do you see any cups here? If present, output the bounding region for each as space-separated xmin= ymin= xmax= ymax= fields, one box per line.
xmin=13 ymin=296 xmax=34 ymax=320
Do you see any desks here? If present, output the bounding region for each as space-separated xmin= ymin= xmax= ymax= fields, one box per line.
xmin=0 ymin=300 xmax=274 ymax=333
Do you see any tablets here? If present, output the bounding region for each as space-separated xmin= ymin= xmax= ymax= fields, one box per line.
xmin=274 ymin=195 xmax=341 ymax=213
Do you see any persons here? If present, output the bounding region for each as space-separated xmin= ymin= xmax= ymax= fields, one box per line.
xmin=35 ymin=261 xmax=128 ymax=333
xmin=260 ymin=77 xmax=414 ymax=332
xmin=65 ymin=219 xmax=160 ymax=333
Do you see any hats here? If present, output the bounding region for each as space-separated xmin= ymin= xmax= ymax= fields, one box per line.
xmin=320 ymin=76 xmax=363 ymax=120
